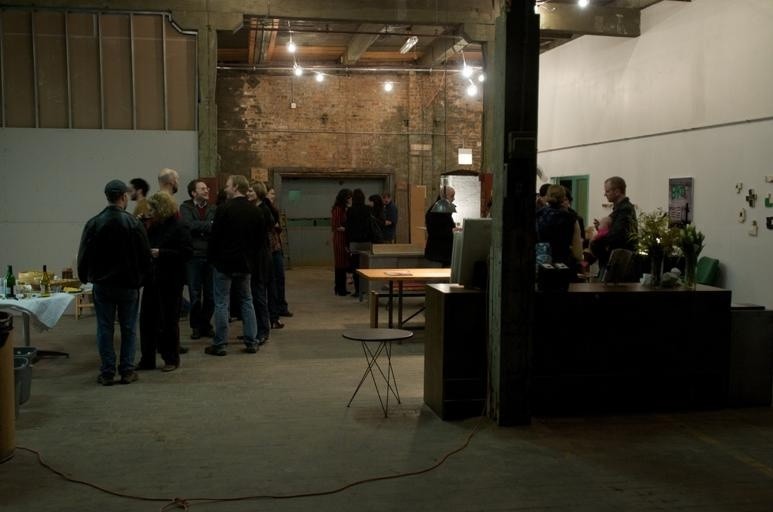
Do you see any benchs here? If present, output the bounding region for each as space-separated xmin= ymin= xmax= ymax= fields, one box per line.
xmin=367 ymin=287 xmax=425 ymax=327
xmin=383 ymin=283 xmax=425 ymax=310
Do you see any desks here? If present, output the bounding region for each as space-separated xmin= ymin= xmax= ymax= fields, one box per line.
xmin=355 ymin=268 xmax=453 ymax=345
xmin=342 ymin=328 xmax=413 ymax=419
xmin=369 ymin=243 xmax=424 ymax=268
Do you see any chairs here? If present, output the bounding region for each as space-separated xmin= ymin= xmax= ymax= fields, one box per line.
xmin=692 ymin=255 xmax=720 ymax=283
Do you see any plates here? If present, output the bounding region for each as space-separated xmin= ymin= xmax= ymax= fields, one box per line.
xmin=60 ymin=288 xmax=84 ymax=293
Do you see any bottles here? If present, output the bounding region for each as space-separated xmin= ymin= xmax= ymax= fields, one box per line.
xmin=40 ymin=265 xmax=50 ymax=297
xmin=62 ymin=264 xmax=73 ymax=281
xmin=5 ymin=264 xmax=16 ymax=299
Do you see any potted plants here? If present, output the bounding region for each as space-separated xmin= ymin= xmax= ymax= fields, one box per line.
xmin=661 ymin=225 xmax=705 ymax=290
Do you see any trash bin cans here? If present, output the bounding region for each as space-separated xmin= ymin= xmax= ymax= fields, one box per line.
xmin=13 ymin=346 xmax=37 ymax=404
xmin=14 ymin=357 xmax=28 ymax=417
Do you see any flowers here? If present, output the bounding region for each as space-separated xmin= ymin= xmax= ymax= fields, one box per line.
xmin=626 ymin=204 xmax=671 ymax=288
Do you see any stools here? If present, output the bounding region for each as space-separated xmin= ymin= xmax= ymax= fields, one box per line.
xmin=73 ymin=291 xmax=93 ymax=321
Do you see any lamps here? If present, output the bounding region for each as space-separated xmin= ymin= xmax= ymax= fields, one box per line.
xmin=457 ymin=104 xmax=473 ymax=165
xmin=399 ymin=36 xmax=419 ymax=55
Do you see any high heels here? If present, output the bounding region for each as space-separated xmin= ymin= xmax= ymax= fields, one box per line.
xmin=335 ymin=289 xmax=350 ymax=296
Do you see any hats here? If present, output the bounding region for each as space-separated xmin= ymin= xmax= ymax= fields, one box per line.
xmin=105 ymin=180 xmax=132 ymax=199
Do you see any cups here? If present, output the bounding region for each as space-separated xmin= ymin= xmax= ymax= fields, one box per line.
xmin=53 ymin=272 xmax=62 ymax=280
xmin=16 ymin=280 xmax=25 ymax=298
xmin=24 ymin=285 xmax=32 ymax=299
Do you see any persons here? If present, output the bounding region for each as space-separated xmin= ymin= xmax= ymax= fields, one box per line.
xmin=486 ymin=197 xmax=493 ymax=217
xmin=133 ymin=191 xmax=188 ymax=371
xmin=535 ymin=177 xmax=638 ymax=281
xmin=332 ymin=188 xmax=398 ymax=298
xmin=424 ymin=187 xmax=455 ymax=266
xmin=206 ymin=175 xmax=268 ymax=356
xmin=126 ymin=168 xmax=291 ymax=353
xmin=77 ymin=180 xmax=151 ymax=386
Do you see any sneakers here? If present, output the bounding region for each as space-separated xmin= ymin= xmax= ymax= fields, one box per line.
xmin=97 ymin=305 xmax=293 ymax=386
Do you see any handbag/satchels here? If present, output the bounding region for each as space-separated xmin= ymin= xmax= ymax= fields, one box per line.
xmin=268 ymin=223 xmax=285 ymax=252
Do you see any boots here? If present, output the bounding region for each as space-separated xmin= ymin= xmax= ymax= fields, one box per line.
xmin=351 ymin=282 xmax=359 ymax=297
